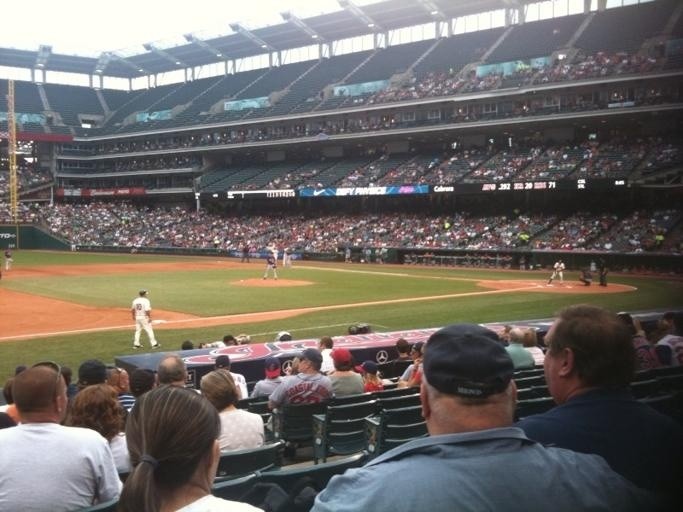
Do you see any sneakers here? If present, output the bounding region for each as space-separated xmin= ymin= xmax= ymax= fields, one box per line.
xmin=151 ymin=342 xmax=162 ymax=350
xmin=132 ymin=344 xmax=146 ymax=349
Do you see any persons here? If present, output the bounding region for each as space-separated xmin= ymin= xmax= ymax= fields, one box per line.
xmin=598 ymin=263 xmax=608 ymax=287
xmin=515 ymin=304 xmax=683 ymax=511
xmin=0 ymin=46 xmax=682 ymax=279
xmin=306 ymin=322 xmax=661 ymax=511
xmin=0 ymin=359 xmax=125 ymax=512
xmin=116 ymin=382 xmax=265 ymax=512
xmin=578 ymin=265 xmax=592 ymax=286
xmin=0 ymin=314 xmax=681 ymax=475
xmin=129 ymin=288 xmax=161 ymax=351
xmin=547 ymin=257 xmax=564 ymax=287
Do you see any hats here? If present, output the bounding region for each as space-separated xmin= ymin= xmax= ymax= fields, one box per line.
xmin=355 ymin=359 xmax=378 ymax=376
xmin=328 ymin=348 xmax=354 ymax=363
xmin=214 ymin=354 xmax=232 ymax=369
xmin=139 ymin=289 xmax=149 ymax=296
xmin=264 ymin=356 xmax=282 ymax=378
xmin=295 ymin=348 xmax=323 ymax=363
xmin=421 ymin=323 xmax=517 ymax=400
xmin=75 ymin=360 xmax=112 ymax=387
xmin=412 ymin=340 xmax=425 ymax=353
